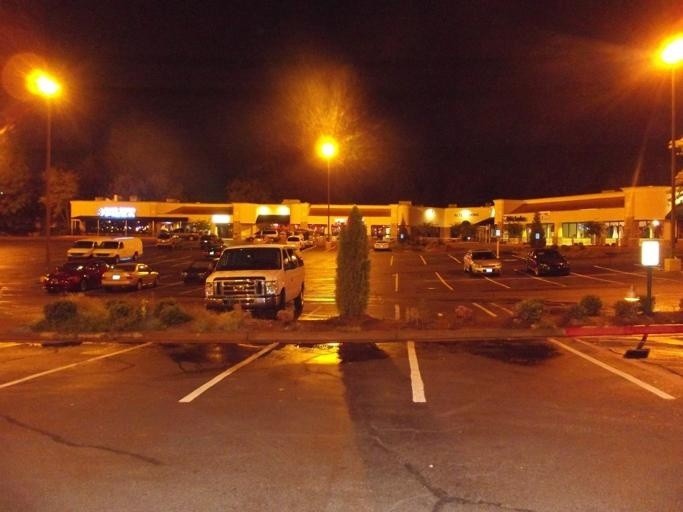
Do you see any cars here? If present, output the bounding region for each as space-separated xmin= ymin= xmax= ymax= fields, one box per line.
xmin=463 ymin=249 xmax=503 ymax=277
xmin=525 ymin=248 xmax=570 ymax=276
xmin=43 ymin=228 xmax=313 ymax=314
xmin=374 ymin=239 xmax=390 ymax=251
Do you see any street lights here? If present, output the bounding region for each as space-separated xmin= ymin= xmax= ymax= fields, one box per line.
xmin=665 ymin=41 xmax=681 ymax=261
xmin=40 ymin=79 xmax=58 ymax=269
xmin=319 ymin=142 xmax=337 ymax=242
